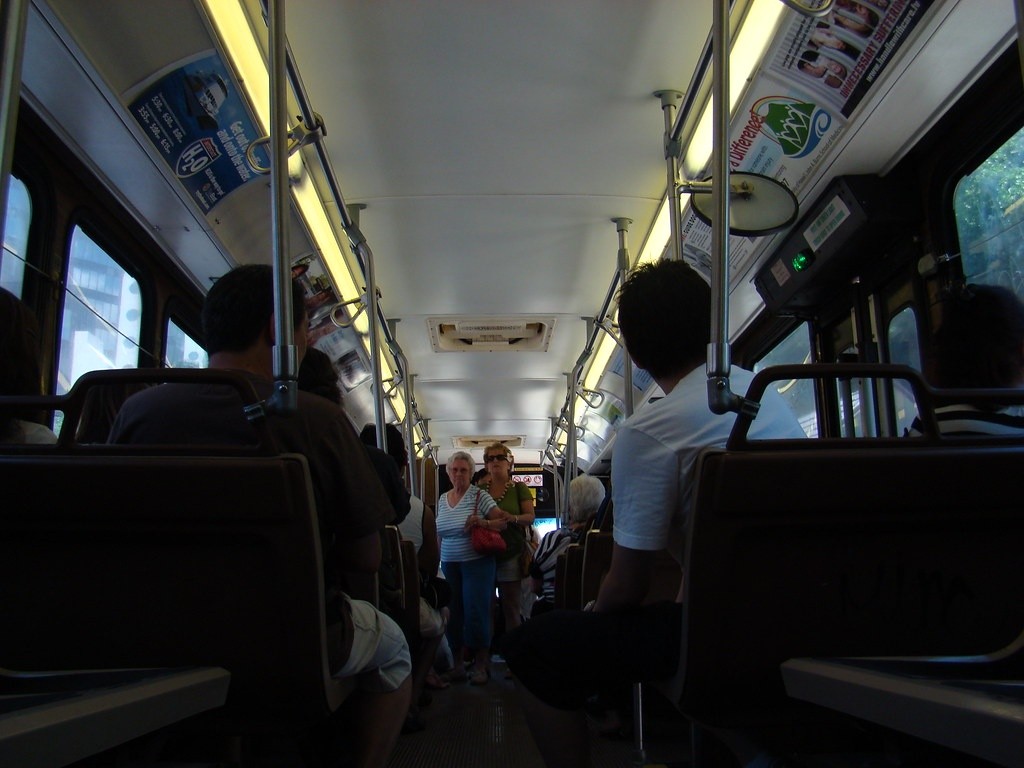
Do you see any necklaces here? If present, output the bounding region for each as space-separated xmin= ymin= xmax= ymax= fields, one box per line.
xmin=486 ymin=477 xmax=511 ymax=504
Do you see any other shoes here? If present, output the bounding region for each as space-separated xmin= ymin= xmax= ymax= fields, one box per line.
xmin=448 ymin=670 xmax=468 ymax=684
xmin=503 ymin=668 xmax=513 ymax=679
xmin=471 ymin=672 xmax=488 ymax=684
xmin=486 ymin=661 xmax=493 ymax=672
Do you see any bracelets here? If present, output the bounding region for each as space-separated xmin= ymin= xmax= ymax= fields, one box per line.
xmin=515 ymin=515 xmax=518 ymax=523
xmin=487 ymin=520 xmax=490 ymax=529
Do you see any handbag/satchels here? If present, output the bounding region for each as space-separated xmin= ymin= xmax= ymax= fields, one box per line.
xmin=472 ymin=490 xmax=506 ymax=553
xmin=516 ymin=541 xmax=533 ymax=577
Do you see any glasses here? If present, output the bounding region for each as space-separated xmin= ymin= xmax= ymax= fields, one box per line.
xmin=486 ymin=454 xmax=509 ymax=462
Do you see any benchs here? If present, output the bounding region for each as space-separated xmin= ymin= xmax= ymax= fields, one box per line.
xmin=554 ymin=495 xmax=614 ymax=612
xmin=0 ymin=368 xmax=362 ymax=767
xmin=347 ymin=525 xmax=427 ymax=659
xmin=632 ymin=362 xmax=1024 ymax=768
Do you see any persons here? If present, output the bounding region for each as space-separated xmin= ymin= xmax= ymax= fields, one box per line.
xmin=902 ymin=283 xmax=1024 ymax=439
xmin=106 ymin=265 xmax=444 ymax=768
xmin=500 ymin=257 xmax=809 ymax=736
xmin=530 ymin=474 xmax=607 ymax=618
xmin=470 ymin=443 xmax=536 ymax=631
xmin=435 ymin=454 xmax=507 ymax=685
xmin=0 ymin=287 xmax=56 ymax=444
